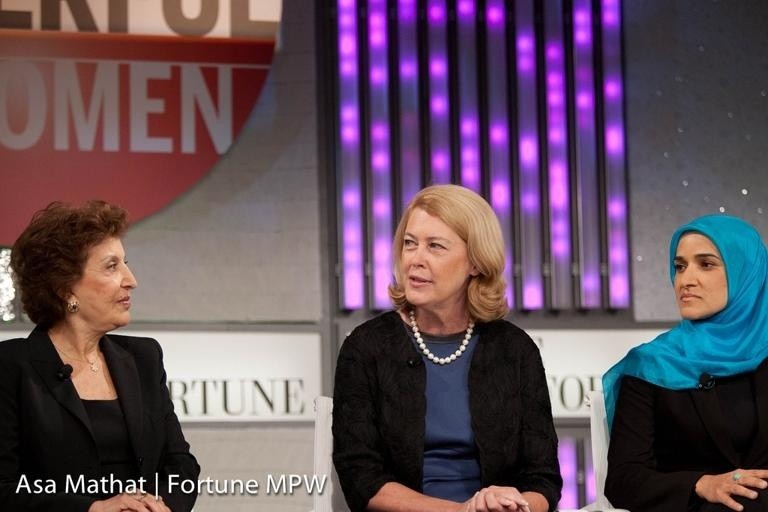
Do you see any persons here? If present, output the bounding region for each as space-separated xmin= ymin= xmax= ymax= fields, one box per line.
xmin=0 ymin=201 xmax=200 ymax=512
xmin=331 ymin=185 xmax=562 ymax=512
xmin=601 ymin=213 xmax=768 ymax=512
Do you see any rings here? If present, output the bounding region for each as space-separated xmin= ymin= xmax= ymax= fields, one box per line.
xmin=138 ymin=492 xmax=147 ymax=501
xmin=732 ymin=473 xmax=742 ymax=481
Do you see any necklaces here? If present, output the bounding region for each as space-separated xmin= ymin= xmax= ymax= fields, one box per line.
xmin=53 ymin=342 xmax=100 ymax=372
xmin=409 ymin=307 xmax=475 ymax=364
